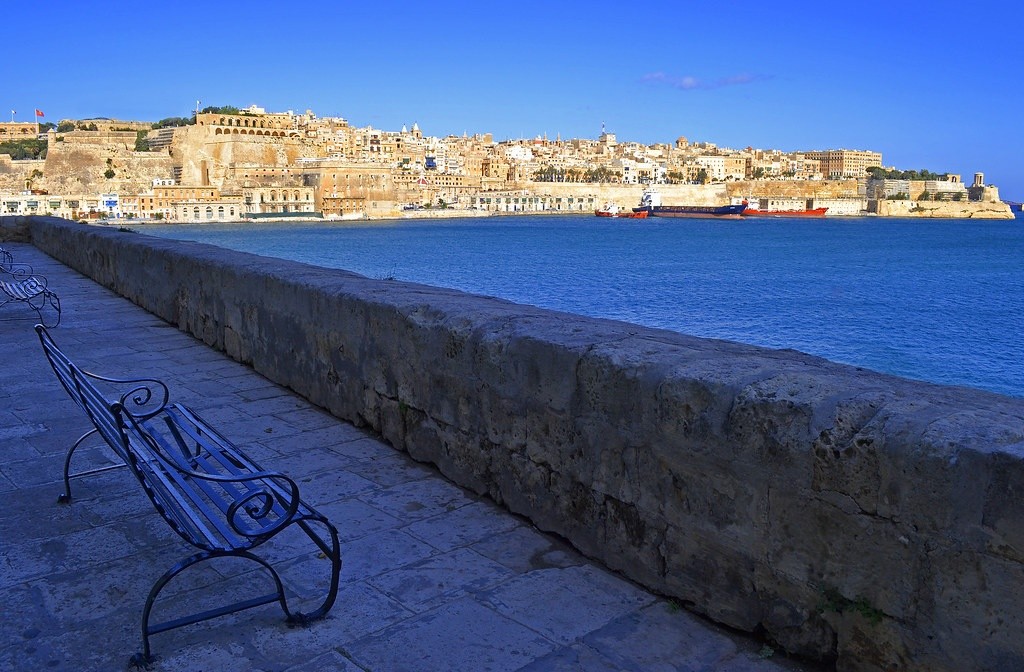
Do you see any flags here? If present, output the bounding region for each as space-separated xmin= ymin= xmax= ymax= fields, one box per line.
xmin=36 ymin=110 xmax=44 ymax=116
xmin=12 ymin=111 xmax=16 ymax=114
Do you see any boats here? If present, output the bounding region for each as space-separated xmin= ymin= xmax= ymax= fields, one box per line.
xmin=632 ymin=203 xmax=748 ymax=221
xmin=615 ymin=211 xmax=649 ymax=220
xmin=741 ymin=206 xmax=830 ymax=219
xmin=594 ymin=209 xmax=614 ymax=217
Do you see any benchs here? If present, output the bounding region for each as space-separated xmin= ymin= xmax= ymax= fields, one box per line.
xmin=35 ymin=324 xmax=344 ymax=672
xmin=0 ymin=247 xmax=61 ymax=328
